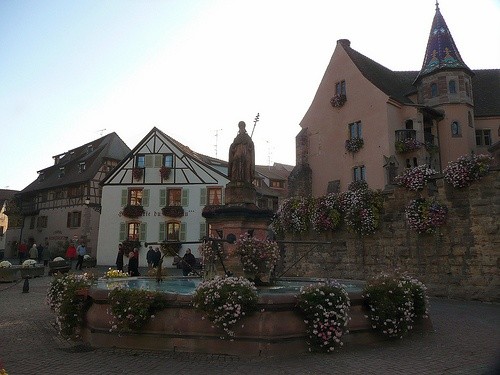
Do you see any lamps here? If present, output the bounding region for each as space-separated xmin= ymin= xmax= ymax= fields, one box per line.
xmin=85 ymin=195 xmax=91 ymax=205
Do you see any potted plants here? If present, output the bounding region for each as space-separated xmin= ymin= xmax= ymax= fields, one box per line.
xmin=48 ymin=257 xmax=67 ymax=268
xmin=20 ymin=259 xmax=44 ymax=279
xmin=0 ymin=261 xmax=20 ymax=282
xmin=82 ymin=255 xmax=96 ymax=267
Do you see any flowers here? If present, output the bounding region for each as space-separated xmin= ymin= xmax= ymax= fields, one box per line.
xmin=330 ymin=94 xmax=344 ymax=108
xmin=45 ymin=136 xmax=492 ymax=354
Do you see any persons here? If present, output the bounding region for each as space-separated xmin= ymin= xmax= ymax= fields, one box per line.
xmin=183 ymin=248 xmax=196 ymax=276
xmin=146 ymin=246 xmax=156 ymax=268
xmin=75 ymin=241 xmax=87 ymax=272
xmin=66 ymin=242 xmax=76 ymax=265
xmin=18 ymin=241 xmax=27 ymax=265
xmin=115 ymin=243 xmax=124 ymax=274
xmin=152 ymin=247 xmax=161 ymax=269
xmin=28 ymin=244 xmax=38 ymax=261
xmin=228 ymin=121 xmax=255 ymax=184
xmin=126 ymin=252 xmax=139 ymax=277
